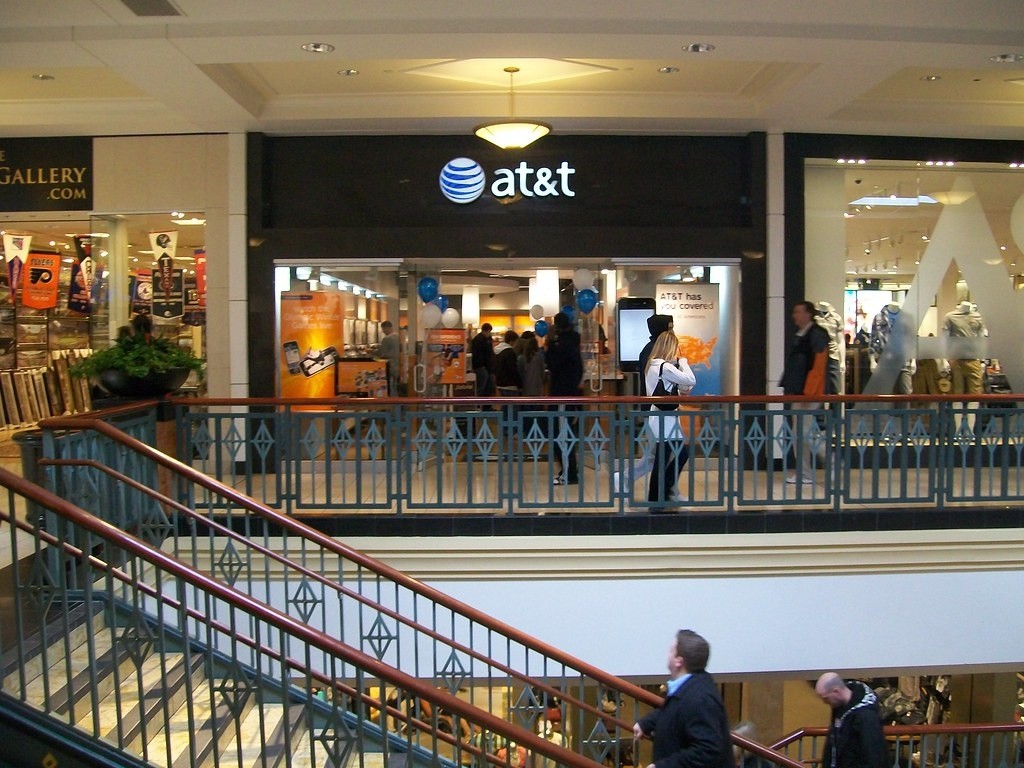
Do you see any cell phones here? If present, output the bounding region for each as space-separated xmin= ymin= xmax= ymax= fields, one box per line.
xmin=283 ymin=341 xmax=340 ymax=378
xmin=616 ymin=296 xmax=657 ymax=373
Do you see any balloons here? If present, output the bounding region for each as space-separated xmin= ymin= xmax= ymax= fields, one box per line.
xmin=560 ymin=305 xmax=574 ymax=321
xmin=535 ymin=320 xmax=549 ymax=337
xmin=574 ymin=269 xmax=592 ymax=291
xmin=441 ymin=308 xmax=459 ymax=328
xmin=531 ymin=304 xmax=544 ymax=320
xmin=418 ymin=277 xmax=437 ymax=303
xmin=576 ymin=289 xmax=596 ymax=314
xmin=431 ymin=294 xmax=447 ymax=313
xmin=422 ymin=304 xmax=440 ymax=328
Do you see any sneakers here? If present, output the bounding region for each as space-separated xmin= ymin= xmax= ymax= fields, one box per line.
xmin=554 ymin=473 xmax=577 ymax=485
xmin=786 ymin=475 xmax=813 ymax=483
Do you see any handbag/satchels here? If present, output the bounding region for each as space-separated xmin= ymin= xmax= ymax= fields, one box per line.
xmin=652 ymin=361 xmax=679 ymax=410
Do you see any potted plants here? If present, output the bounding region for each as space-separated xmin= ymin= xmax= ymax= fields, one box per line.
xmin=66 ymin=320 xmax=205 ymax=396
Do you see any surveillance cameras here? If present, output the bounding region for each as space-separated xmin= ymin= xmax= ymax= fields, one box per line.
xmin=854 ymin=179 xmax=861 ymax=184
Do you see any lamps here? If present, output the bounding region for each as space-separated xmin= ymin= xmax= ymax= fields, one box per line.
xmin=473 ymin=67 xmax=552 ymax=151
xmin=863 ymin=233 xmax=921 ymax=272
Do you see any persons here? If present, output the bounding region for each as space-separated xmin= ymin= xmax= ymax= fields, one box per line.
xmin=813 ymin=302 xmax=845 ymax=444
xmin=778 ymin=302 xmax=845 ymax=482
xmin=527 ymin=720 xmax=567 ymax=768
xmin=116 ymin=315 xmax=155 ymax=346
xmin=868 ymin=302 xmax=916 ymax=441
xmin=470 ymin=311 xmax=696 ymax=513
xmin=942 ymin=301 xmax=989 ymax=439
xmin=844 ymin=333 xmax=950 ymax=383
xmin=365 ymin=320 xmax=400 ymax=396
xmin=815 ymin=672 xmax=889 ymax=768
xmin=633 ymin=630 xmax=735 ymax=768
xmin=495 ymin=742 xmax=526 ymax=768
